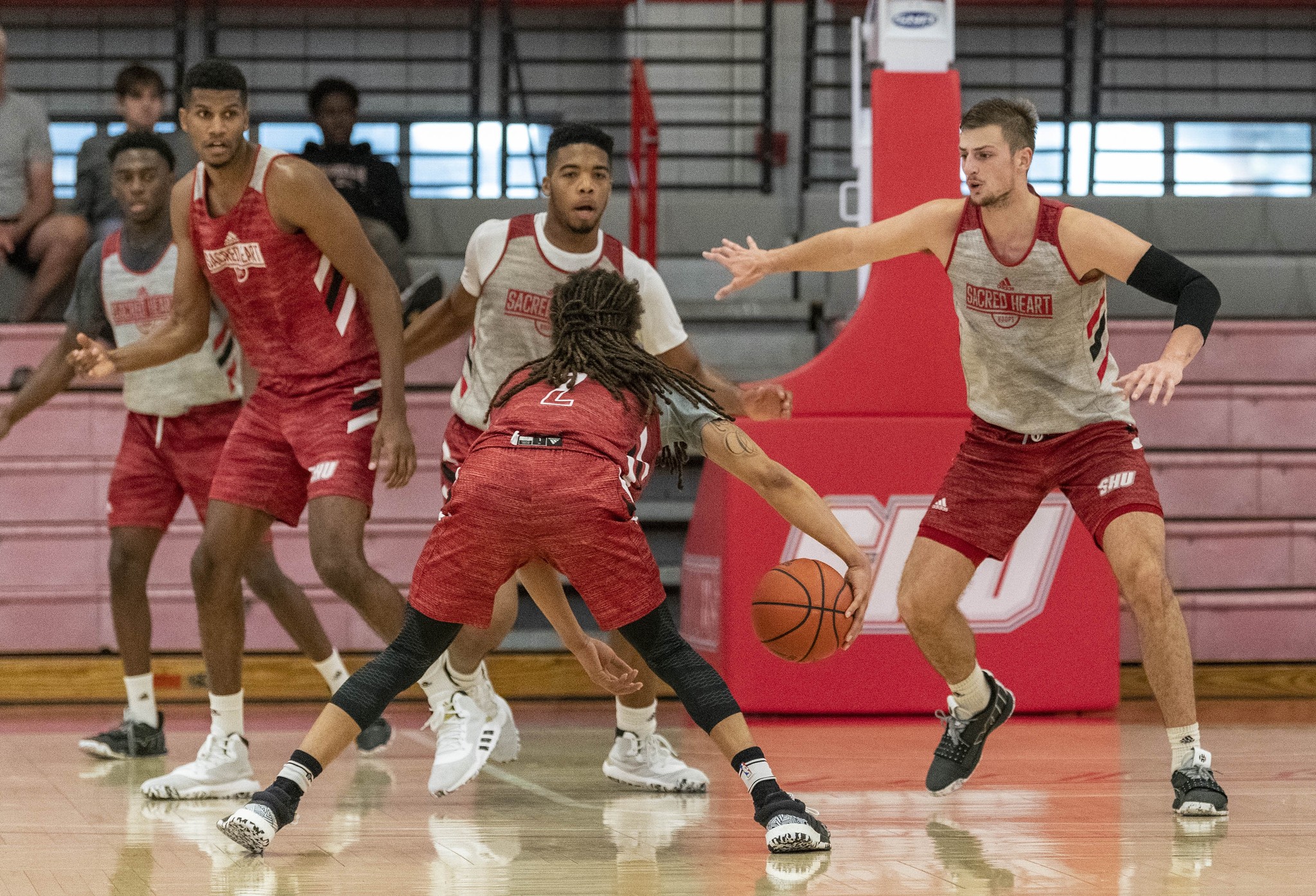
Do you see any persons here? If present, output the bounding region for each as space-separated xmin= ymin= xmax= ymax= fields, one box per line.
xmin=704 ymin=95 xmax=1235 ymax=818
xmin=0 ymin=29 xmax=90 ymax=323
xmin=75 ymin=66 xmax=202 ymax=250
xmin=215 ymin=265 xmax=873 ymax=855
xmin=68 ymin=60 xmax=503 ymax=803
xmin=403 ymin=124 xmax=792 ymax=790
xmin=78 ymin=762 xmax=1235 ymax=896
xmin=0 ymin=128 xmax=394 ymax=761
xmin=283 ymin=74 xmax=444 ymax=323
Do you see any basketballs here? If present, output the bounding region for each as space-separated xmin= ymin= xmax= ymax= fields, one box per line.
xmin=752 ymin=558 xmax=854 ymax=663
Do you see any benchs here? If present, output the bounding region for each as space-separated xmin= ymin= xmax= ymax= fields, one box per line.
xmin=1103 ymin=321 xmax=1316 ymax=663
xmin=1 ymin=320 xmax=470 ymax=655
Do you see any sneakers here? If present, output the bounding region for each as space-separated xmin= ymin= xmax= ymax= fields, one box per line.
xmin=761 ymin=802 xmax=831 ymax=855
xmin=924 ymin=666 xmax=1016 ymax=799
xmin=77 ymin=710 xmax=168 ymax=757
xmin=470 ymin=661 xmax=520 ymax=764
xmin=429 ymin=812 xmax=522 ymax=874
xmin=353 ymin=718 xmax=393 ymax=755
xmin=140 ymin=733 xmax=262 ymax=798
xmin=142 ymin=790 xmax=258 ymax=853
xmin=603 ymin=783 xmax=709 ymax=850
xmin=766 ymin=848 xmax=830 ymax=890
xmin=602 ymin=732 xmax=708 ymax=794
xmin=418 ymin=692 xmax=501 ymax=798
xmin=217 ymin=796 xmax=277 ymax=855
xmin=1170 ymin=749 xmax=1228 ymax=819
